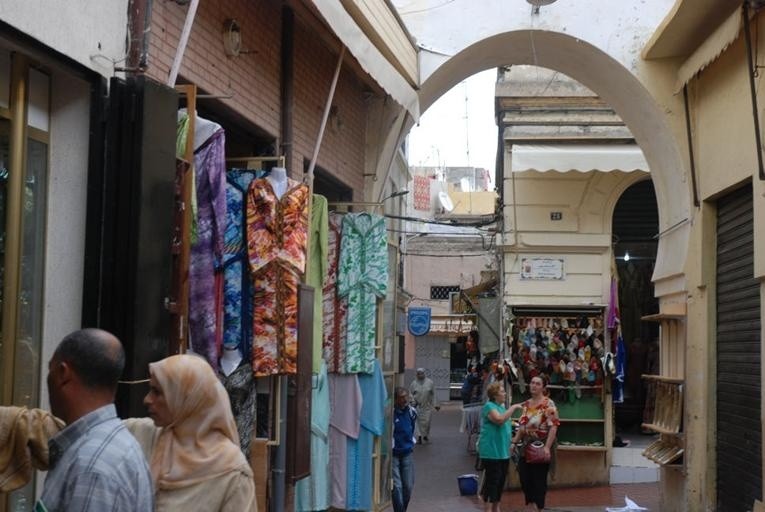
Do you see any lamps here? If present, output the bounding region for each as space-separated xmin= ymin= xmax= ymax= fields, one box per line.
xmin=379 ymin=189 xmax=410 ymax=206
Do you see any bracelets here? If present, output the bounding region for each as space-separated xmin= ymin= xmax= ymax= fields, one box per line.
xmin=511 ymin=442 xmax=517 ymax=445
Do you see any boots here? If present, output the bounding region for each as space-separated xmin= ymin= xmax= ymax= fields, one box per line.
xmin=613 ymin=436 xmax=629 ymax=447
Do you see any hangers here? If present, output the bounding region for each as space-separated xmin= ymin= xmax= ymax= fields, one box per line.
xmin=356 ymin=202 xmax=372 ymax=220
xmin=330 ymin=201 xmax=350 ymax=216
xmin=235 ymin=158 xmax=255 ymax=175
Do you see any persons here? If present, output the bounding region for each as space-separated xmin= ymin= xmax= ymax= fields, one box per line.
xmin=173 ymin=107 xmax=229 ymax=270
xmin=460 ymin=365 xmax=486 ymax=456
xmin=33 ymin=326 xmax=155 ymax=512
xmin=474 ymin=380 xmax=525 ymax=512
xmin=389 ymin=387 xmax=419 ymax=512
xmin=119 ymin=352 xmax=259 ymax=512
xmin=508 ymin=374 xmax=561 ymax=512
xmin=245 ymin=167 xmax=312 ymax=276
xmin=407 ymin=367 xmax=440 ymax=444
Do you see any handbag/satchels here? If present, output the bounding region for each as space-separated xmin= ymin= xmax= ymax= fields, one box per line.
xmin=525 ymin=440 xmax=545 ymax=464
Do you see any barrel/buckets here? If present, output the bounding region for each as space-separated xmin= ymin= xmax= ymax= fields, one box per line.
xmin=456 ymin=475 xmax=479 ymax=496
